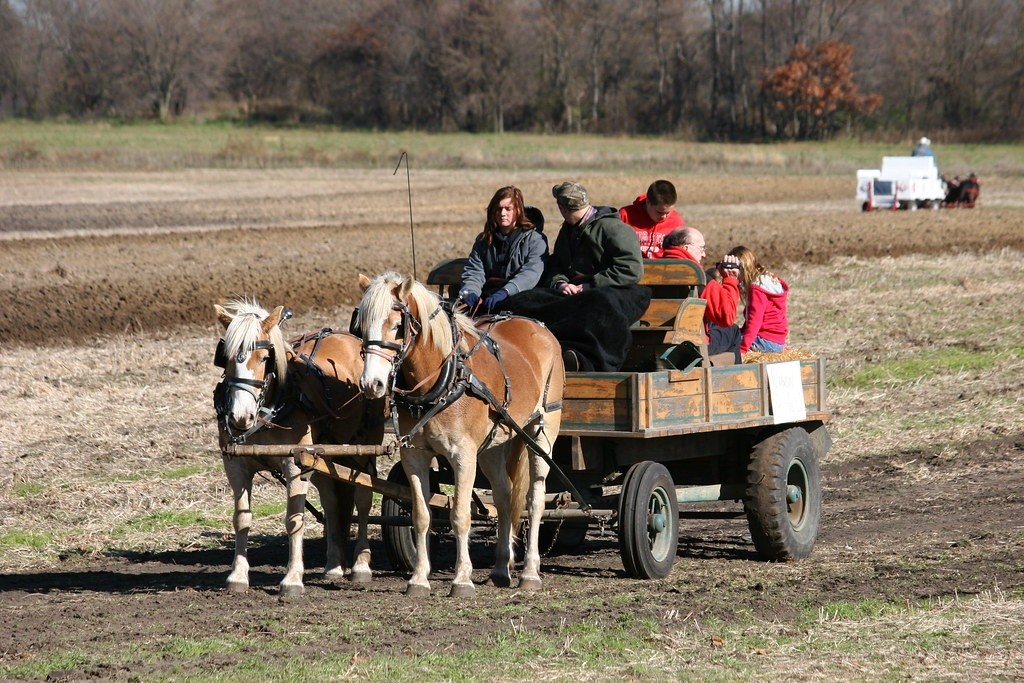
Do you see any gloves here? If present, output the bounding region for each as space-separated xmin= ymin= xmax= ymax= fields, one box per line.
xmin=462 ymin=293 xmax=478 ymax=306
xmin=483 ymin=288 xmax=509 ymax=310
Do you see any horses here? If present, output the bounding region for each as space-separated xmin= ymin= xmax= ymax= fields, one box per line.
xmin=353 ymin=269 xmax=566 ymax=599
xmin=211 ymin=288 xmax=387 ymax=599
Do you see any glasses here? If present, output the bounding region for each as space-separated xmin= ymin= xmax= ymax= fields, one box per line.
xmin=685 ymin=242 xmax=707 ymax=252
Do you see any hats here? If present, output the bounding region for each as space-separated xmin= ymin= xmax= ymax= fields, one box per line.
xmin=552 ymin=182 xmax=590 ymax=210
xmin=919 ymin=137 xmax=931 ymax=145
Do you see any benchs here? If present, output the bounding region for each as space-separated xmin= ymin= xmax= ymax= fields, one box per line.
xmin=427 ymin=259 xmax=706 ymax=371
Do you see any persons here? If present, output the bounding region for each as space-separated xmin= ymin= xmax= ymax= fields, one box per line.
xmin=547 ymin=180 xmax=643 ymax=372
xmin=620 ymin=180 xmax=788 ymax=365
xmin=459 ymin=185 xmax=546 ymax=313
xmin=912 ymin=136 xmax=934 ymax=156
xmin=522 ymin=206 xmax=549 ymax=260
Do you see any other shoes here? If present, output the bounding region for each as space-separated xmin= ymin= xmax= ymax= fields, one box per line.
xmin=562 ymin=349 xmax=581 ymax=371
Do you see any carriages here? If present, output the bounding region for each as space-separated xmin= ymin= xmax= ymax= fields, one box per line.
xmin=211 ymin=257 xmax=835 ymax=600
xmin=852 ymin=155 xmax=980 ymax=211
